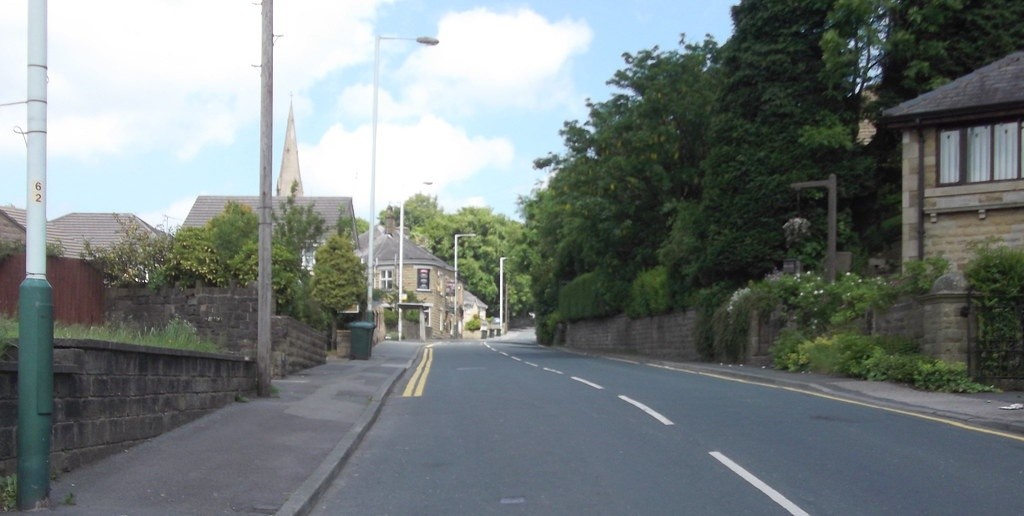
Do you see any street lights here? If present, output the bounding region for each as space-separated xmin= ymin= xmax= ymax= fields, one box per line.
xmin=364 ymin=35 xmax=440 ymax=320
xmin=397 ymin=179 xmax=434 ymax=343
xmin=453 ymin=233 xmax=478 ymax=343
xmin=499 ymin=256 xmax=522 ymax=336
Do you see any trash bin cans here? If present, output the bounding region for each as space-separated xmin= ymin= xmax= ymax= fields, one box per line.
xmin=348 ymin=321 xmax=377 ymax=361
xmin=496 ymin=328 xmax=501 ymax=336
xmin=481 ymin=329 xmax=487 ymax=339
xmin=489 ymin=328 xmax=495 ymax=338
